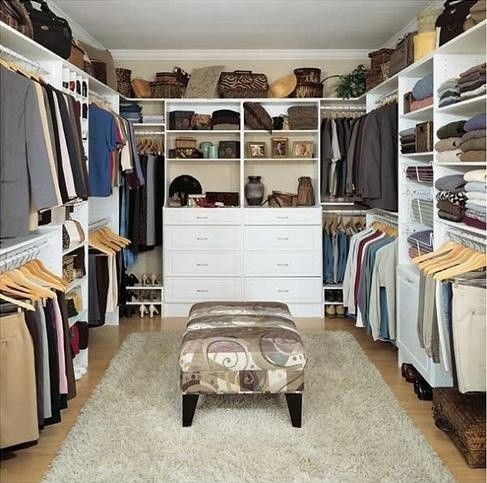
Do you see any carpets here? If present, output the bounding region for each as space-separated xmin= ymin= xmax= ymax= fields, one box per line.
xmin=43 ymin=330 xmax=456 ymax=481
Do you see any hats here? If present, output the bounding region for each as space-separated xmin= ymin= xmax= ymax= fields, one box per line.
xmin=268 ymin=74 xmax=297 ymax=97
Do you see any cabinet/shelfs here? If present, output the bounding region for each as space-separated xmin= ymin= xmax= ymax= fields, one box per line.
xmin=130 ymin=64 xmax=320 ymax=326
xmin=0 ymin=0 xmax=131 ymax=455
xmin=321 ymin=0 xmax=486 ymax=475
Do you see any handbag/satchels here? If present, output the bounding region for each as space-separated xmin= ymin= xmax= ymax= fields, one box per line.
xmin=217 ymin=70 xmax=268 ymax=97
xmin=389 ymin=30 xmax=418 ymax=78
xmin=17 ymin=0 xmax=72 ymax=59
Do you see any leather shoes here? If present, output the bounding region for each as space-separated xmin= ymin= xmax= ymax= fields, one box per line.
xmin=402 ymin=362 xmax=433 ymax=399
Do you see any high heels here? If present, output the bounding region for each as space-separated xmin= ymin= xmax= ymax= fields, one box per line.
xmin=122 ymin=273 xmax=159 ymax=318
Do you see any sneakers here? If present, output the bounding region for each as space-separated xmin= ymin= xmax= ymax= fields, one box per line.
xmin=324 ymin=289 xmax=346 ymax=319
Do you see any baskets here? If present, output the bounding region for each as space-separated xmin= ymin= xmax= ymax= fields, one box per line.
xmin=431 ymin=386 xmax=485 ymax=467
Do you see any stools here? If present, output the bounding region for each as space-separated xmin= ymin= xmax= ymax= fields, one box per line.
xmin=178 ymin=302 xmax=307 ymax=427
